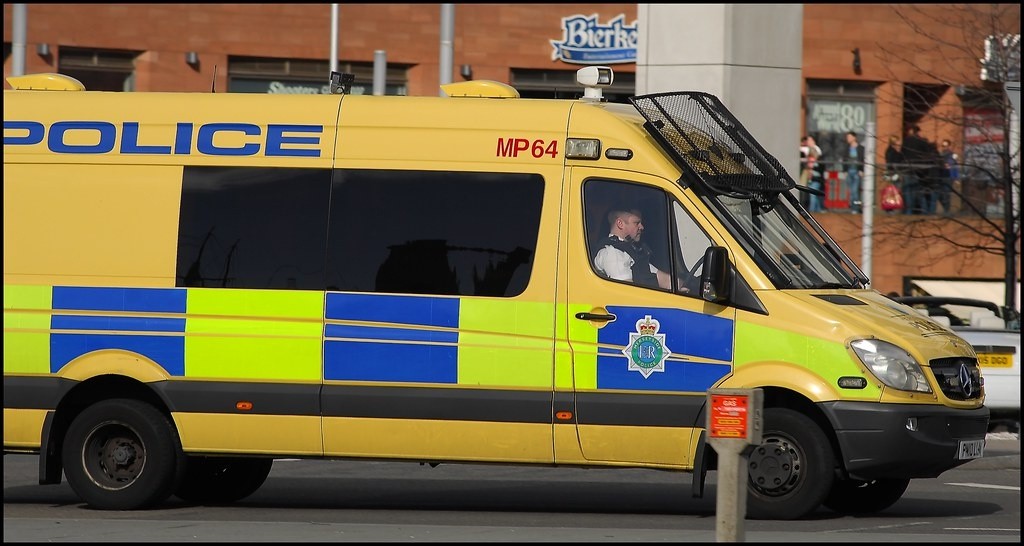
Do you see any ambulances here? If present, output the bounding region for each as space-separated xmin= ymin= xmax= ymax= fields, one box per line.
xmin=2 ymin=65 xmax=988 ymax=524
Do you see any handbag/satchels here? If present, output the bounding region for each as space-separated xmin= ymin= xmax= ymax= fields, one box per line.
xmin=882 ymin=185 xmax=903 ymax=208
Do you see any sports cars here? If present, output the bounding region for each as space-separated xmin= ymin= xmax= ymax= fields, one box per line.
xmin=881 ymin=295 xmax=1023 ymax=445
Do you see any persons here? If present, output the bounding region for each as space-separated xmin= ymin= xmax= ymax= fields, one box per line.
xmin=841 ymin=131 xmax=864 ymax=214
xmin=799 ymin=135 xmax=826 ymax=212
xmin=593 ymin=204 xmax=692 ymax=294
xmin=885 ymin=121 xmax=940 ymax=215
xmin=937 ymin=139 xmax=960 ymax=214
